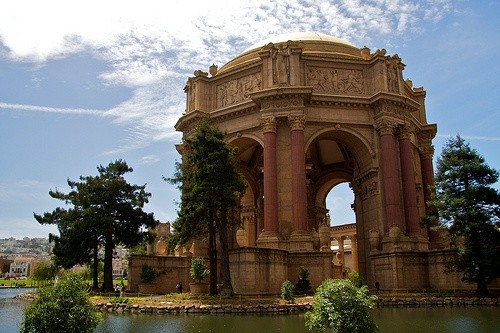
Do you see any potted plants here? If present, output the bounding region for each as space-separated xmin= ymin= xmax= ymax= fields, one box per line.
xmin=188 ymin=256 xmax=211 ymax=295
xmin=279 ymin=281 xmax=295 ymax=304
xmin=136 ymin=263 xmax=160 ymax=294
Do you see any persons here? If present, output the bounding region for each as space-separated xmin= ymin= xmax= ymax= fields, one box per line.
xmin=386 ymin=60 xmax=398 ymax=92
xmin=219 ymin=75 xmax=260 ymax=104
xmin=272 ymin=43 xmax=290 ymax=85
xmin=374 ymin=279 xmax=380 ymax=294
xmin=176 ymin=280 xmax=183 ymax=294
xmin=309 ymin=69 xmax=362 ymax=94
xmin=120 ymin=283 xmax=125 ymax=297
xmin=114 ymin=284 xmax=120 ymax=298
